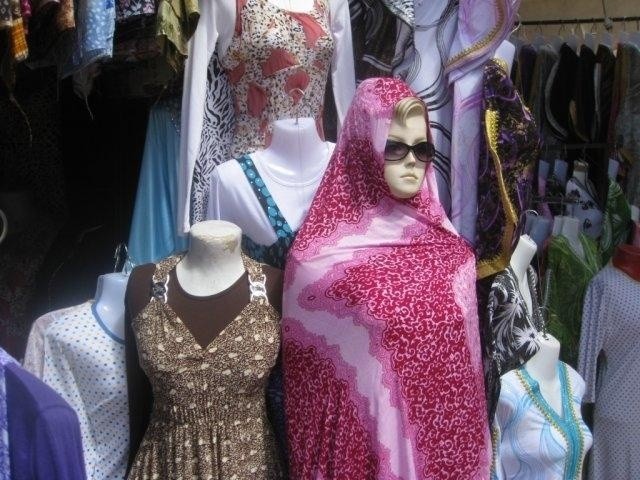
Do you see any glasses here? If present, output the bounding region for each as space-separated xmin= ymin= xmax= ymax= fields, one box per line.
xmin=385 ymin=140 xmax=436 ymax=162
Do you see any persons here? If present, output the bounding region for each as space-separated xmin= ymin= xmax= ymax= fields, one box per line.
xmin=0 ymin=0 xmax=640 ymax=480
